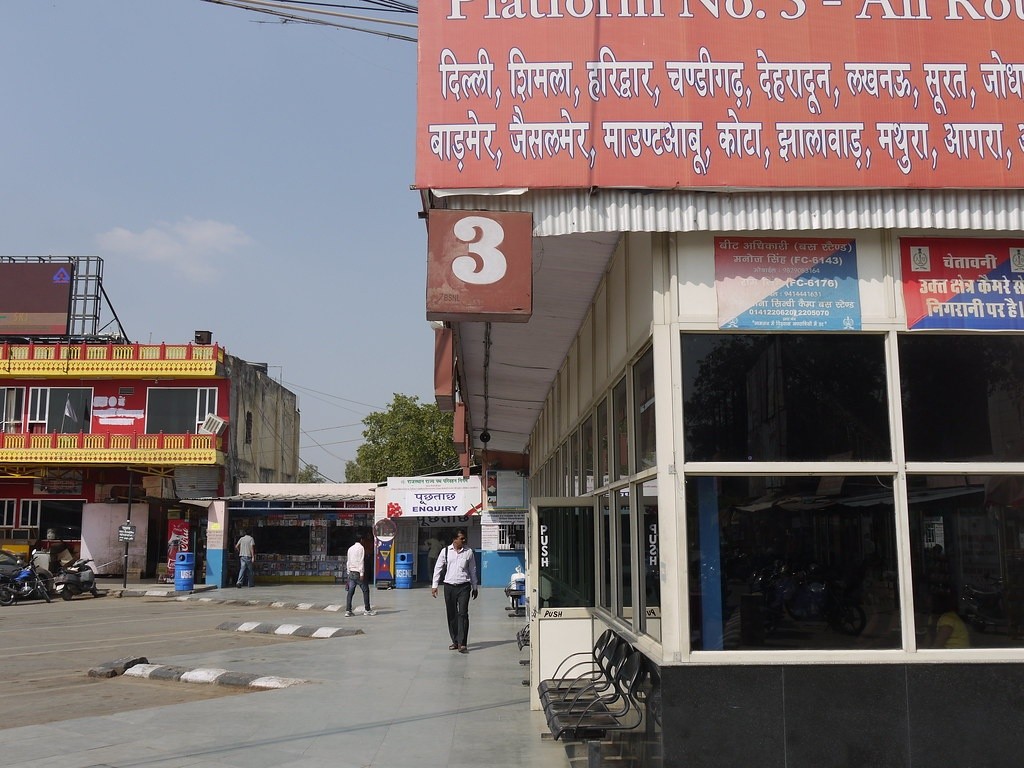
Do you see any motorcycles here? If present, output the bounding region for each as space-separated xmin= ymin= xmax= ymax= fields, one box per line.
xmin=0 ymin=549 xmax=52 ymax=607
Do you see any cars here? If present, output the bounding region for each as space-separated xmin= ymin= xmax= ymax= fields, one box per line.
xmin=1 ymin=549 xmax=54 ymax=595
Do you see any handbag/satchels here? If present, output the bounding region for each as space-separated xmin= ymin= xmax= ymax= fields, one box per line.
xmin=439 ymin=564 xmax=447 ymax=585
xmin=345 ymin=575 xmax=350 ymax=590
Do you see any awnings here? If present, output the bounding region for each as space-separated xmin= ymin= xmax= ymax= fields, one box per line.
xmin=188 ymin=491 xmax=375 ymax=504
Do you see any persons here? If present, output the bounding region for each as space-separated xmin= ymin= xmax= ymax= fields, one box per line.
xmin=425 ymin=532 xmax=446 ymax=585
xmin=846 ymin=564 xmax=900 ymax=647
xmin=430 ymin=529 xmax=479 ymax=654
xmin=344 ymin=536 xmax=378 ymax=617
xmin=922 ymin=583 xmax=972 ymax=649
xmin=235 ymin=528 xmax=254 ymax=588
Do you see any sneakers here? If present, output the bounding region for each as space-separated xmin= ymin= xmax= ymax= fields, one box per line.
xmin=364 ymin=610 xmax=378 ymax=616
xmin=344 ymin=612 xmax=355 ymax=617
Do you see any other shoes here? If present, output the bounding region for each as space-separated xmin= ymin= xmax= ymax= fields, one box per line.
xmin=236 ymin=583 xmax=241 ymax=588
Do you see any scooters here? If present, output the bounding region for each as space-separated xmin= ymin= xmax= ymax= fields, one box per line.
xmin=55 ymin=558 xmax=99 ymax=601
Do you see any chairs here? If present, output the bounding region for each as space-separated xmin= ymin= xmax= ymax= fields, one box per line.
xmin=535 ymin=627 xmax=647 ymax=766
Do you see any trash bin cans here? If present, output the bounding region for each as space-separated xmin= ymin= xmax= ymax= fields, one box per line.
xmin=175 ymin=552 xmax=194 ymax=591
xmin=394 ymin=553 xmax=414 ymax=589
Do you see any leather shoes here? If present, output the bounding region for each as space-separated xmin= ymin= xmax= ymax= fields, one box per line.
xmin=450 ymin=643 xmax=467 ymax=652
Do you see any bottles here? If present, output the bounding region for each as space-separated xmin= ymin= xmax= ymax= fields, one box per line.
xmin=168 ymin=528 xmax=183 ymax=575
